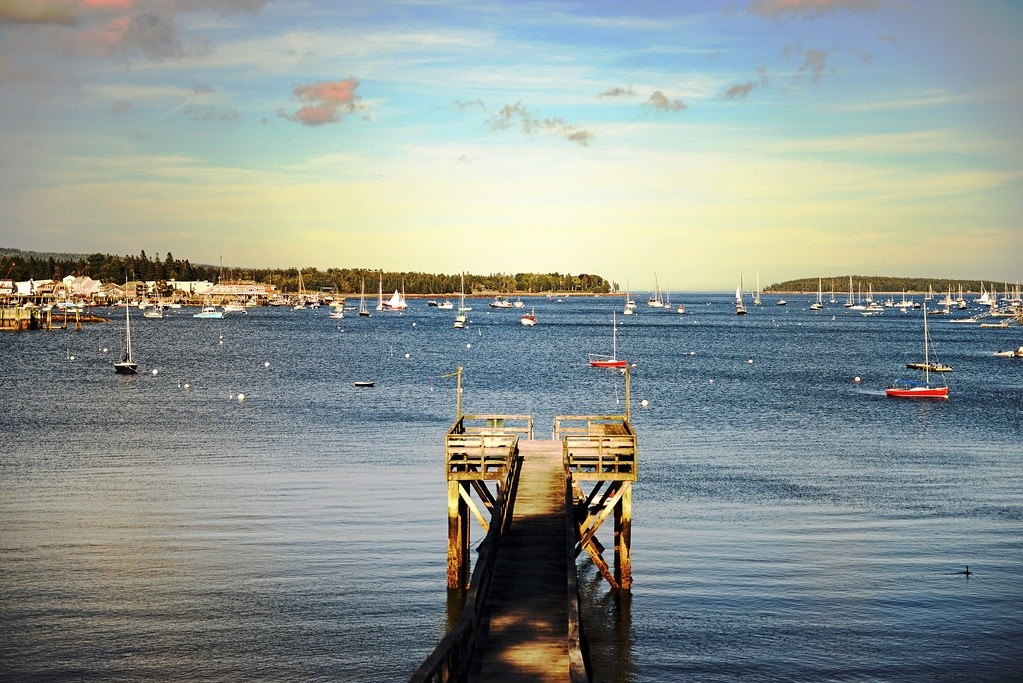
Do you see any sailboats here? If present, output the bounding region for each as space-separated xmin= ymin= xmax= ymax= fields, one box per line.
xmin=753 ymin=271 xmax=1023 ymax=329
xmin=456 ymin=272 xmax=514 ymax=311
xmin=143 ymin=288 xmax=163 ymax=318
xmin=113 ymin=275 xmax=137 ymax=373
xmin=736 ymin=274 xmax=747 ymax=315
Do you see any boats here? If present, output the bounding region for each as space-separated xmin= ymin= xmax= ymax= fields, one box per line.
xmin=624 ymin=274 xmax=685 ymax=316
xmin=514 ymin=302 xmax=524 ymax=308
xmin=907 ymin=303 xmax=952 ymax=372
xmin=886 ymin=387 xmax=948 ymax=398
xmin=265 ymin=273 xmax=409 ymax=319
xmin=427 ymin=298 xmax=468 ymax=330
xmin=994 ymin=346 xmax=1022 ymax=357
xmin=521 ymin=315 xmax=537 ymax=326
xmin=590 ymin=310 xmax=626 ymax=369
xmin=223 ymin=300 xmax=245 ymax=314
xmin=192 ymin=306 xmax=223 ymax=319
